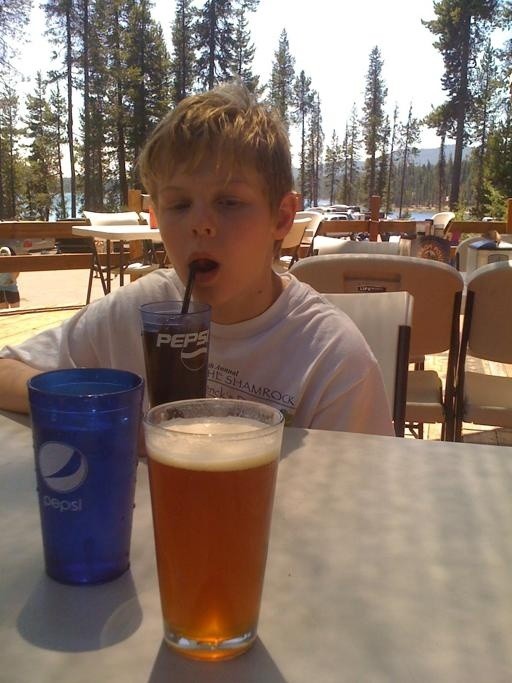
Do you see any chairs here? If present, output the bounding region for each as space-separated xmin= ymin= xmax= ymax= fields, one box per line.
xmin=0 ymin=209 xmax=512 ymax=442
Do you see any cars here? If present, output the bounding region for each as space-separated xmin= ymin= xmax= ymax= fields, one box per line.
xmin=306 ymin=204 xmax=364 ymax=238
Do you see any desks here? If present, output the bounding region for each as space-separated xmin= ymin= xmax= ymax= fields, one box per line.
xmin=2 ymin=399 xmax=511 ymax=681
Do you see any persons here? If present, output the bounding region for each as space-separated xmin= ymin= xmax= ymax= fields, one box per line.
xmin=0 ymin=78 xmax=400 ymax=458
xmin=0 ymin=245 xmax=20 ymax=310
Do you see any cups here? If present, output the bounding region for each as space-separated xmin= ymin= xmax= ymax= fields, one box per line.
xmin=141 ymin=396 xmax=287 ymax=665
xmin=139 ymin=300 xmax=212 ymax=405
xmin=27 ymin=367 xmax=145 ymax=593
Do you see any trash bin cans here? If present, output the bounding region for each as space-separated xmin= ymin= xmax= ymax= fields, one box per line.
xmin=56 ymin=218 xmax=97 ymax=254
